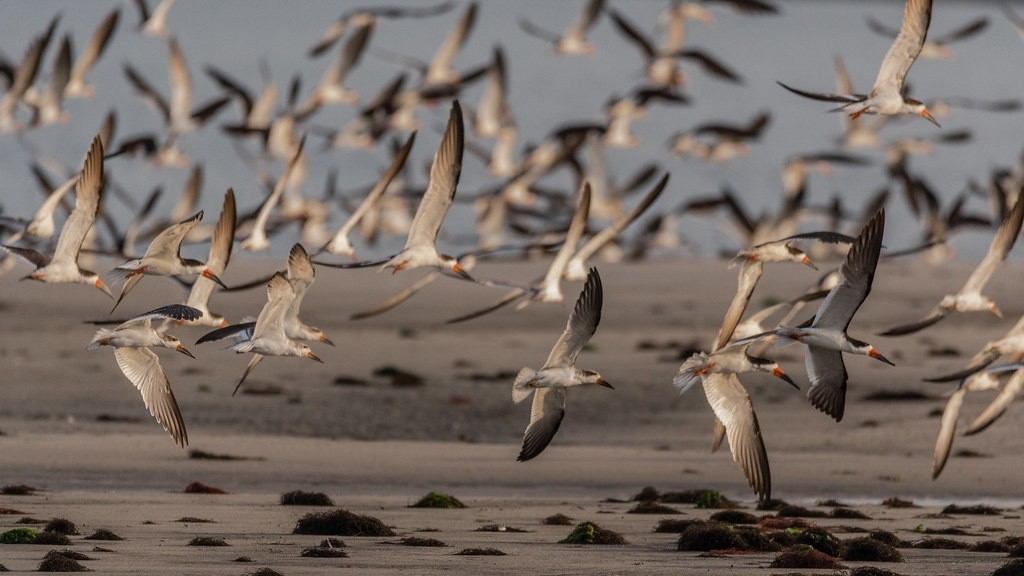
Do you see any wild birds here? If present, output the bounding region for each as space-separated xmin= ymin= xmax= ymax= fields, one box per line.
xmin=0 ymin=0 xmax=1024 ymax=513
